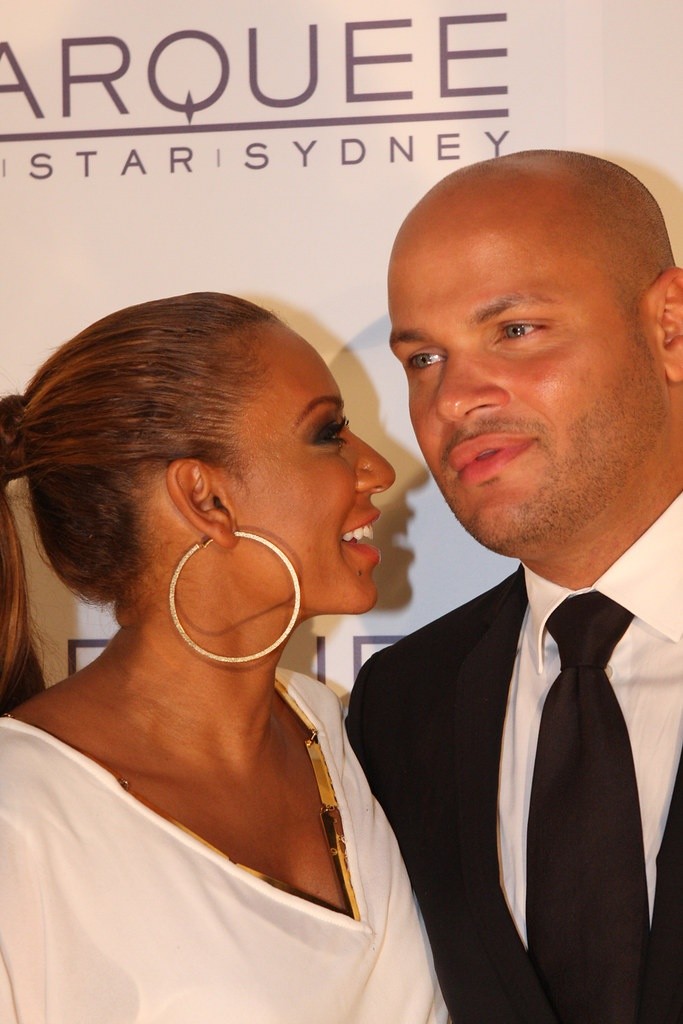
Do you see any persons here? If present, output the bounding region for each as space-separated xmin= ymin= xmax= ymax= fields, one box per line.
xmin=1 ymin=290 xmax=452 ymax=1024
xmin=346 ymin=145 xmax=683 ymax=1024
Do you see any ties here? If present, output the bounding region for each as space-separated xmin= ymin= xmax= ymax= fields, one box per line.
xmin=527 ymin=591 xmax=651 ymax=1024
